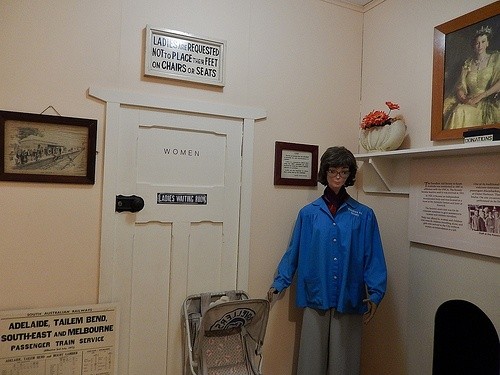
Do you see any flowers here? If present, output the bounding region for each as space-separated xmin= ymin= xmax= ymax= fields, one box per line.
xmin=360 ymin=101 xmax=400 ymax=130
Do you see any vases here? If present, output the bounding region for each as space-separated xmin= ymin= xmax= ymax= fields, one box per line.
xmin=361 ymin=120 xmax=408 ymax=153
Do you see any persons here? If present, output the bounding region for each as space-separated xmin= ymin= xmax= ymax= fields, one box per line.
xmin=266 ymin=145 xmax=388 ymax=375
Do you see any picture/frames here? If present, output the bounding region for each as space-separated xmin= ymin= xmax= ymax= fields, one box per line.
xmin=144 ymin=23 xmax=227 ymax=88
xmin=274 ymin=141 xmax=319 ymax=187
xmin=0 ymin=110 xmax=98 ymax=185
xmin=430 ymin=0 xmax=500 ymax=141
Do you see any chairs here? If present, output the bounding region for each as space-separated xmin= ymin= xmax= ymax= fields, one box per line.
xmin=183 ymin=290 xmax=270 ymax=375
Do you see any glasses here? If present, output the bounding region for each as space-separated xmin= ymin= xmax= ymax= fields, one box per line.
xmin=326 ymin=169 xmax=351 ymax=179
